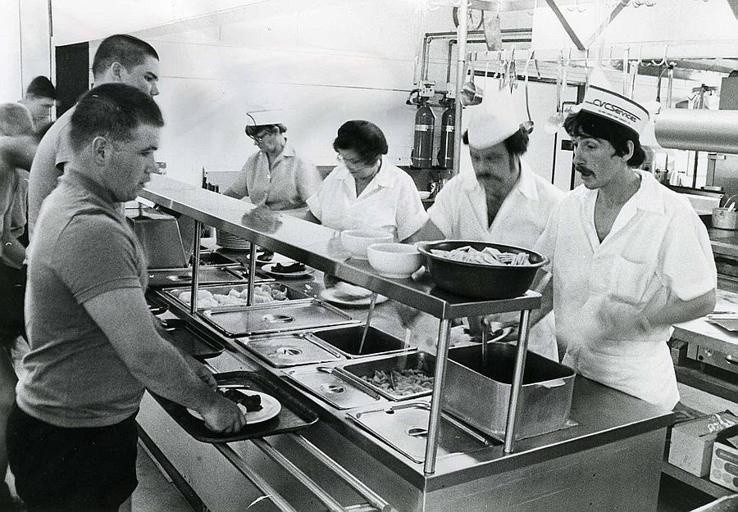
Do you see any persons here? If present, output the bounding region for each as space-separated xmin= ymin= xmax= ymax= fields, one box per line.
xmin=220 ymin=108 xmax=322 ymax=221
xmin=300 ymin=118 xmax=428 ymax=246
xmin=482 ymin=79 xmax=720 ymax=419
xmin=392 ymin=99 xmax=567 ymax=364
xmin=18 ymin=75 xmax=56 ymax=133
xmin=1 ymin=101 xmax=35 ymax=311
xmin=4 ymin=83 xmax=247 ymax=512
xmin=27 ymin=33 xmax=163 ymax=243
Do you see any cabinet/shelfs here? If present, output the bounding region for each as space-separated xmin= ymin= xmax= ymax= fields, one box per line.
xmin=128 ymin=171 xmax=680 ymax=511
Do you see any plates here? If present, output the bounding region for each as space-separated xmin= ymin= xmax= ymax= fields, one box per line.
xmin=320 ymin=285 xmax=389 ymax=308
xmin=436 ymin=322 xmax=514 ymax=350
xmin=213 ymin=228 xmax=260 ymax=250
xmin=260 ymin=262 xmax=317 ymax=278
xmin=186 ymin=389 xmax=282 ymax=426
xmin=246 ymin=251 xmax=297 ymax=264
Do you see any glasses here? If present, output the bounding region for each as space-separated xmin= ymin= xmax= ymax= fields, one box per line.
xmin=250 ymin=133 xmax=271 ymax=142
xmin=335 ymin=154 xmax=364 ymax=166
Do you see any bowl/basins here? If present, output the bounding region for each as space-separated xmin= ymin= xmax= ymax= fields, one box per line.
xmin=435 ymin=344 xmax=576 ymax=442
xmin=417 ymin=240 xmax=551 ymax=301
xmin=304 ymin=323 xmax=415 ymax=359
xmin=341 ymin=229 xmax=394 ymax=260
xmin=336 ymin=349 xmax=443 ymax=401
xmin=367 ymin=243 xmax=424 ymax=279
xmin=160 ymin=280 xmax=313 ymax=315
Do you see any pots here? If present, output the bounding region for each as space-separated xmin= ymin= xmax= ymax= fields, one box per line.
xmin=712 ymin=208 xmax=738 ymax=231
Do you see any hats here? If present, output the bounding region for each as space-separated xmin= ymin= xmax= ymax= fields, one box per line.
xmin=243 ymin=108 xmax=285 ymax=126
xmin=569 ymin=85 xmax=650 ymax=136
xmin=465 ymin=93 xmax=529 ymax=151
